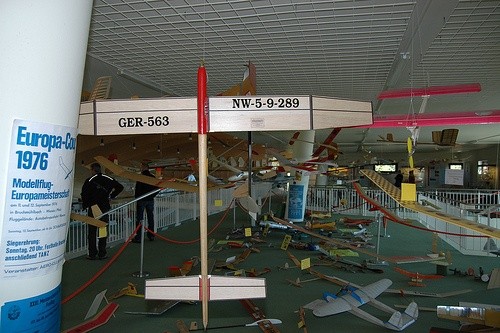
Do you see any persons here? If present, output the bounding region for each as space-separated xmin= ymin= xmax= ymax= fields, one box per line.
xmin=131 ymin=162 xmax=160 ymax=243
xmin=80 ymin=163 xmax=124 ymax=260
xmin=409 ymin=171 xmax=415 ymax=183
xmin=394 ymin=170 xmax=403 ymax=187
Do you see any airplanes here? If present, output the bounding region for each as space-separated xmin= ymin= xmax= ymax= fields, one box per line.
xmin=75 ymin=61 xmax=374 ymax=331
xmin=345 ymin=81 xmax=500 ymax=145
xmin=311 ymin=277 xmax=420 ymax=332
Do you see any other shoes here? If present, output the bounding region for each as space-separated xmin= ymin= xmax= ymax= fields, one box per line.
xmin=129 ymin=240 xmax=141 ymax=243
xmin=150 ymin=236 xmax=154 ymax=241
xmin=87 ymin=255 xmax=96 ymax=260
xmin=98 ymin=254 xmax=108 ymax=260
